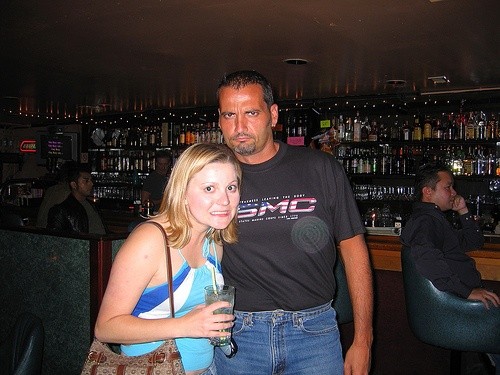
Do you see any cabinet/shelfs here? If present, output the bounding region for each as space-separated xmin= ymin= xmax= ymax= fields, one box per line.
xmin=82 ymin=91 xmax=500 ymax=243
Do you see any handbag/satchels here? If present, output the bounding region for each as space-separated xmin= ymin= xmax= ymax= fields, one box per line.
xmin=80 ymin=338 xmax=186 ymax=375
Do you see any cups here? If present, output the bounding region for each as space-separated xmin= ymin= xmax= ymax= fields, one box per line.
xmin=363 ymin=205 xmax=394 ymax=228
xmin=204 ymin=284 xmax=234 ymax=347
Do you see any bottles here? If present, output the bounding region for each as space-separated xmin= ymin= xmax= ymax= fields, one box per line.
xmin=86 ymin=108 xmax=500 ymax=202
xmin=2 ymin=136 xmax=20 ymax=153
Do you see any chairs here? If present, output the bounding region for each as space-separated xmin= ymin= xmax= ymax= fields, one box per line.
xmin=0 ymin=310 xmax=45 ymax=375
xmin=401 ymin=246 xmax=500 ymax=375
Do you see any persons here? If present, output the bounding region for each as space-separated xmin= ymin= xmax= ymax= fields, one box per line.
xmin=36 ymin=160 xmax=74 ymax=226
xmin=212 ymin=70 xmax=374 ymax=375
xmin=94 ymin=142 xmax=242 ymax=375
xmin=47 ymin=163 xmax=110 ymax=234
xmin=400 ymin=164 xmax=500 ymax=375
